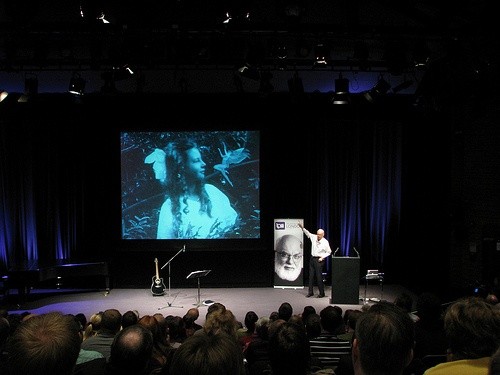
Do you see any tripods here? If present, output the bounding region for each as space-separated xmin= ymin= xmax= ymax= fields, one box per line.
xmin=158 ymin=249 xmax=183 ymax=310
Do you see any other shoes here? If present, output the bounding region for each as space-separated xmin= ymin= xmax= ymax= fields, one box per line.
xmin=307 ymin=293 xmax=314 ymax=297
xmin=317 ymin=295 xmax=325 ymax=298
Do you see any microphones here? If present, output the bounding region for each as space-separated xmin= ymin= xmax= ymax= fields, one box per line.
xmin=334 ymin=248 xmax=339 ymax=257
xmin=183 ymin=245 xmax=185 ymax=252
xmin=354 ymin=247 xmax=359 ymax=257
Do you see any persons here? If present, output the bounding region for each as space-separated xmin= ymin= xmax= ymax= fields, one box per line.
xmin=0 ymin=293 xmax=500 ymax=375
xmin=156 ymin=138 xmax=237 ymax=239
xmin=298 ymin=223 xmax=332 ymax=298
xmin=274 ymin=235 xmax=303 ymax=286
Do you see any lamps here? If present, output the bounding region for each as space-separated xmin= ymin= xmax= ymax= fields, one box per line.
xmin=67 ymin=70 xmax=88 ymax=95
xmin=331 ymin=71 xmax=351 ymax=106
xmin=14 ymin=72 xmax=39 ymax=103
xmin=364 ymin=71 xmax=391 ymax=102
xmin=287 ymin=70 xmax=305 ymax=96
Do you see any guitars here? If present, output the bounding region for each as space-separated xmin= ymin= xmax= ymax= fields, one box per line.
xmin=151 ymin=257 xmax=166 ymax=296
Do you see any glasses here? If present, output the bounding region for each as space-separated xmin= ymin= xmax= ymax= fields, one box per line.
xmin=275 ymin=250 xmax=302 ymax=259
